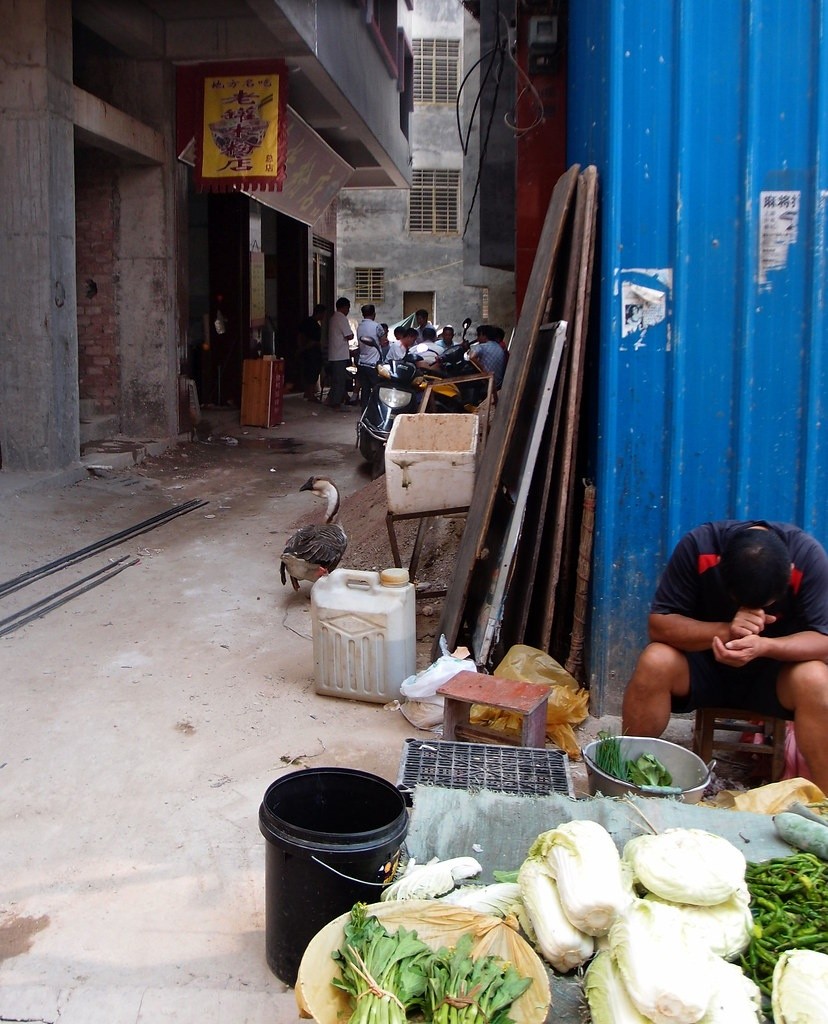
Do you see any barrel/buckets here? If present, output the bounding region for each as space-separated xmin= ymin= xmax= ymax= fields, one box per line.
xmin=258 ymin=766 xmax=409 ymax=988
xmin=310 ymin=568 xmax=417 ymax=703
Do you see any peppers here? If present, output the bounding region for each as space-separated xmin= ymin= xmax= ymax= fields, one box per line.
xmin=739 ymin=854 xmax=828 ymax=1023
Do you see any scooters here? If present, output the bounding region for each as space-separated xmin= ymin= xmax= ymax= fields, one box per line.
xmin=354 ymin=335 xmax=448 ymax=477
xmin=400 ymin=317 xmax=491 ymax=421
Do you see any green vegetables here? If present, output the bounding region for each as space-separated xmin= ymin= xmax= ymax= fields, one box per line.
xmin=331 ymin=900 xmax=534 ymax=1024
xmin=629 ymin=753 xmax=673 ymax=788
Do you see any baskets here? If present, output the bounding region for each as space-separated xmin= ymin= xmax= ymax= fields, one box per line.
xmin=396 ymin=736 xmax=575 ymax=807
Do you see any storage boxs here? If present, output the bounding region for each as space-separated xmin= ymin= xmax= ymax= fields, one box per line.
xmin=385 ymin=413 xmax=479 ymax=515
xmin=395 ymin=737 xmax=576 ymax=807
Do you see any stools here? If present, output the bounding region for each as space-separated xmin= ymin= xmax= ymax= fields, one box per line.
xmin=436 ymin=671 xmax=553 ymax=748
xmin=693 ymin=708 xmax=786 ymax=782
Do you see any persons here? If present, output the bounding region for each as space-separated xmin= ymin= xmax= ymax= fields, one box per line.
xmin=324 ymin=297 xmax=354 ymax=410
xmin=357 ymin=305 xmax=389 ymax=416
xmin=469 ymin=325 xmax=507 ymax=393
xmin=435 ymin=327 xmax=460 ymax=349
xmin=296 ymin=305 xmax=327 ymax=403
xmin=621 ymin=520 xmax=827 ymax=797
xmin=394 ymin=326 xmax=406 ymax=340
xmin=408 ymin=328 xmax=446 ymax=366
xmin=384 ymin=328 xmax=419 ymax=363
xmin=414 ymin=309 xmax=437 ymax=345
xmin=380 ymin=324 xmax=390 ymax=361
xmin=493 ymin=327 xmax=510 ymax=363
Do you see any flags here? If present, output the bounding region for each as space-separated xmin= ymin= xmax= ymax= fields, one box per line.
xmin=193 ymin=59 xmax=286 ymax=192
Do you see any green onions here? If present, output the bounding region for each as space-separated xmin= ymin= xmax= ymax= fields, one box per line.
xmin=593 ymin=724 xmax=632 ymax=786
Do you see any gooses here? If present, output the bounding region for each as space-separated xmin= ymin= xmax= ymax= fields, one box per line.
xmin=280 ymin=475 xmax=350 ymax=601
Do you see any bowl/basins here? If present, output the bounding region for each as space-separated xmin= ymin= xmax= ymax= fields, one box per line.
xmin=582 ymin=737 xmax=711 ymax=803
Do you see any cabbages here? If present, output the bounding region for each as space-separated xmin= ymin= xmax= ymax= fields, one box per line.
xmin=383 ymin=817 xmax=828 ymax=1024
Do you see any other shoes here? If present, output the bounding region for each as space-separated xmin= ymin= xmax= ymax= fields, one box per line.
xmin=324 ymin=400 xmax=351 ymax=412
